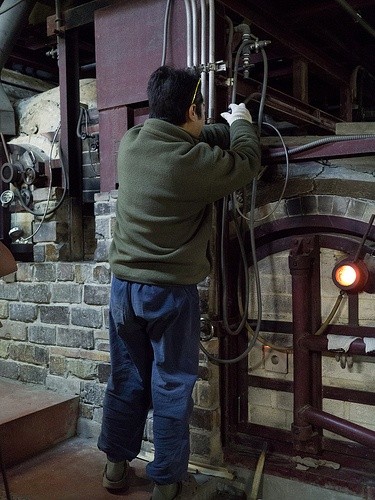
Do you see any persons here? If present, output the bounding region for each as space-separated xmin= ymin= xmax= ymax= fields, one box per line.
xmin=98 ymin=66 xmax=258 ymax=499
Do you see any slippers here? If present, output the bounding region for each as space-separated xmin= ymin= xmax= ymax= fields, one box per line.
xmin=150 ymin=473 xmax=198 ymax=500
xmin=102 ymin=460 xmax=129 ymax=489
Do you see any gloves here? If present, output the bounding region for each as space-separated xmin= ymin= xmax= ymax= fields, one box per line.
xmin=327 ymin=334 xmax=362 ymax=369
xmin=363 ymin=337 xmax=375 ymax=355
xmin=221 ymin=103 xmax=252 ymax=125
